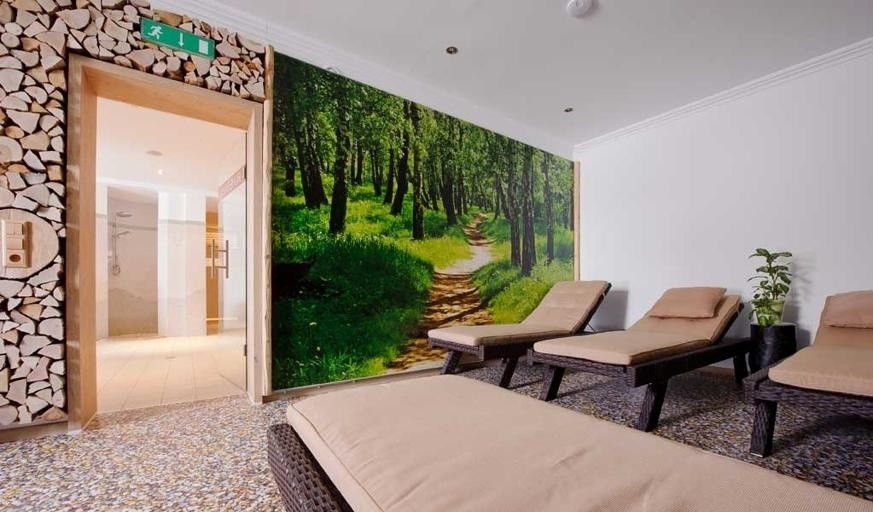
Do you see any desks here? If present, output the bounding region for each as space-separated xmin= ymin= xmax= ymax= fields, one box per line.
xmin=749 ymin=322 xmax=796 ymax=373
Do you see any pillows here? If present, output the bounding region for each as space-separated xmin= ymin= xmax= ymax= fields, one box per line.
xmin=648 ymin=286 xmax=727 ymax=318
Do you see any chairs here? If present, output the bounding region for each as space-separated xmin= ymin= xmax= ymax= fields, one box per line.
xmin=428 ymin=280 xmax=610 ymax=387
xmin=264 ymin=375 xmax=872 ymax=512
xmin=527 ymin=285 xmax=750 ymax=432
xmin=743 ymin=290 xmax=873 ymax=458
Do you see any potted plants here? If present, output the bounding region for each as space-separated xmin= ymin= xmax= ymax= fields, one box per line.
xmin=747 ymin=247 xmax=794 ymax=327
